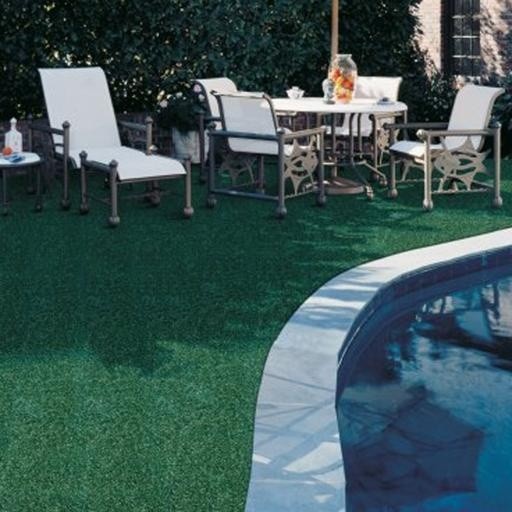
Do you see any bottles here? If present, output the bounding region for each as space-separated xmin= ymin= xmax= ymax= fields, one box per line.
xmin=4 ymin=117 xmax=24 ymax=156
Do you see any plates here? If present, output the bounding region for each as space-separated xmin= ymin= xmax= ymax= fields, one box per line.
xmin=375 ymin=99 xmax=396 ymax=106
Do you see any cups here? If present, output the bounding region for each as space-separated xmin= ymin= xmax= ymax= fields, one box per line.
xmin=286 ymin=88 xmax=305 ymax=102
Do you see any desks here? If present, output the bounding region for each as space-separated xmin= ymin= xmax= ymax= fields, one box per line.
xmin=1 ymin=150 xmax=46 ymax=217
xmin=260 ymin=94 xmax=409 ymax=201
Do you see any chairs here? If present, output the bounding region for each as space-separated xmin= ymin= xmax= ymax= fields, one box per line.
xmin=317 ymin=71 xmax=409 ymax=190
xmin=205 ymin=88 xmax=332 ymax=217
xmin=381 ymin=82 xmax=509 ymax=214
xmin=23 ymin=65 xmax=198 ymax=228
xmin=188 ymin=73 xmax=297 ymax=190
